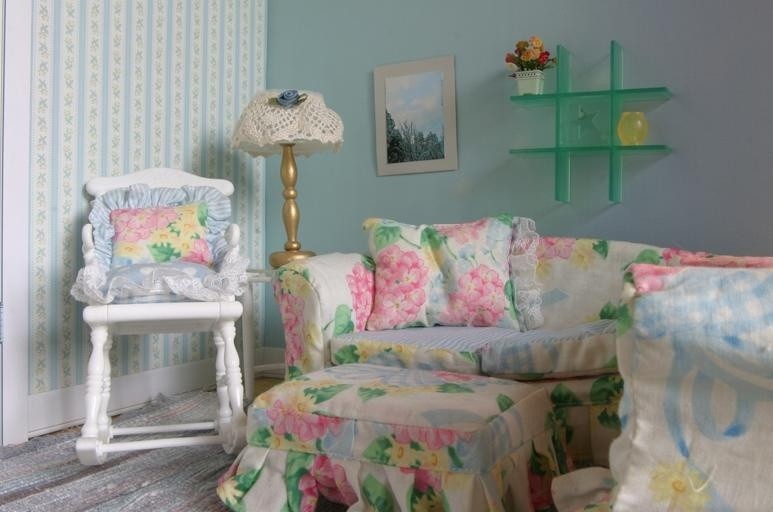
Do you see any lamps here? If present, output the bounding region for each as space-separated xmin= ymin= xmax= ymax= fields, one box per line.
xmin=232 ymin=88 xmax=343 ymax=269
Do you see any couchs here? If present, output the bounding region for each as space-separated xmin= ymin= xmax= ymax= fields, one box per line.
xmin=272 ymin=237 xmax=773 ymax=512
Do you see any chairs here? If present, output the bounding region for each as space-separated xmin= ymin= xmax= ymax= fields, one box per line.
xmin=74 ymin=170 xmax=250 ymax=468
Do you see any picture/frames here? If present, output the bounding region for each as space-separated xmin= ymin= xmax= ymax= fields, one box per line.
xmin=373 ymin=54 xmax=458 ymax=176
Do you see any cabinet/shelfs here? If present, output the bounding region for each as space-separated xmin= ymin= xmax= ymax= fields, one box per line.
xmin=508 ymin=41 xmax=673 ymax=202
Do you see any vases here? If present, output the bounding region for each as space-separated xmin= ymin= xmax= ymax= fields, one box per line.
xmin=516 ymin=70 xmax=545 ymax=95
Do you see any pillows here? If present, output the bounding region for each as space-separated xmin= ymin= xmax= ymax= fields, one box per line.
xmin=363 ymin=214 xmax=542 ymax=333
xmin=70 ymin=184 xmax=247 ymax=301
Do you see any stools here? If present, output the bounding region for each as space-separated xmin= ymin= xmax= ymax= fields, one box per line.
xmin=215 ymin=364 xmax=573 ymax=512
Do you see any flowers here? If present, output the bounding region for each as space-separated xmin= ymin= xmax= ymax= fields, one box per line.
xmin=503 ymin=35 xmax=556 ymax=75
xmin=269 ymin=91 xmax=307 ymax=107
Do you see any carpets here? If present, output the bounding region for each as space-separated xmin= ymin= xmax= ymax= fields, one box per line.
xmin=1 ymin=386 xmax=270 ymax=512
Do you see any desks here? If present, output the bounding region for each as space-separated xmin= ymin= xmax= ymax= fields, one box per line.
xmin=236 ymin=269 xmax=282 ymax=405
